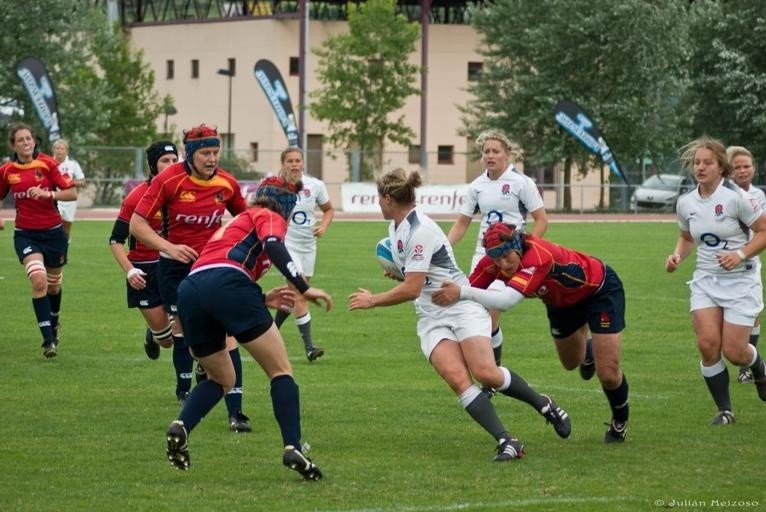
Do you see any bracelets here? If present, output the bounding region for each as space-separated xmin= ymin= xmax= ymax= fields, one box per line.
xmin=736 ymin=249 xmax=746 ymax=261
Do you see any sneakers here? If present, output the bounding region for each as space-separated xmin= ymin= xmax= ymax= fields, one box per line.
xmin=581 ymin=340 xmax=597 ymax=379
xmin=143 ymin=329 xmax=163 ymax=361
xmin=753 ymin=379 xmax=766 ymax=396
xmin=482 ymin=385 xmax=498 ymax=399
xmin=604 ymin=421 xmax=627 ymax=444
xmin=42 ymin=340 xmax=57 ymax=357
xmin=283 ymin=449 xmax=322 ymax=480
xmin=538 ymin=394 xmax=572 ymax=438
xmin=166 ymin=422 xmax=192 ymax=471
xmin=305 ymin=344 xmax=324 ymax=362
xmin=712 ymin=410 xmax=736 ymax=428
xmin=493 ymin=438 xmax=528 ymax=462
xmin=231 ymin=416 xmax=255 ymax=434
xmin=735 ymin=367 xmax=752 ymax=386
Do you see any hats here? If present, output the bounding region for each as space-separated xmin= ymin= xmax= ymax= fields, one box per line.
xmin=255 ymin=176 xmax=299 ymax=217
xmin=145 ymin=142 xmax=178 ymax=174
xmin=180 ymin=124 xmax=221 ymax=179
xmin=481 ymin=224 xmax=523 ymax=259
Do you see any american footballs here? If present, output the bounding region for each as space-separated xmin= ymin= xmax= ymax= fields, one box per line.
xmin=375 ymin=238 xmax=404 ymax=282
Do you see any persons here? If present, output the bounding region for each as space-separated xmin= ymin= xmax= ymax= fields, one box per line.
xmin=128 ymin=126 xmax=250 ymax=408
xmin=0 ymin=123 xmax=78 ymax=357
xmin=245 ymin=148 xmax=333 ymax=362
xmin=167 ymin=177 xmax=333 ymax=470
xmin=724 ymin=144 xmax=766 ymax=384
xmin=444 ymin=129 xmax=548 ymax=400
xmin=110 ymin=142 xmax=178 ymax=360
xmin=51 ymin=140 xmax=84 ymax=241
xmin=346 ymin=169 xmax=571 ymax=464
xmin=431 ymin=223 xmax=630 ymax=444
xmin=665 ymin=140 xmax=766 ymax=420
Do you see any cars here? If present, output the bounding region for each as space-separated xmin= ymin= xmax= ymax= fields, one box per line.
xmin=630 ymin=173 xmax=695 ymax=215
